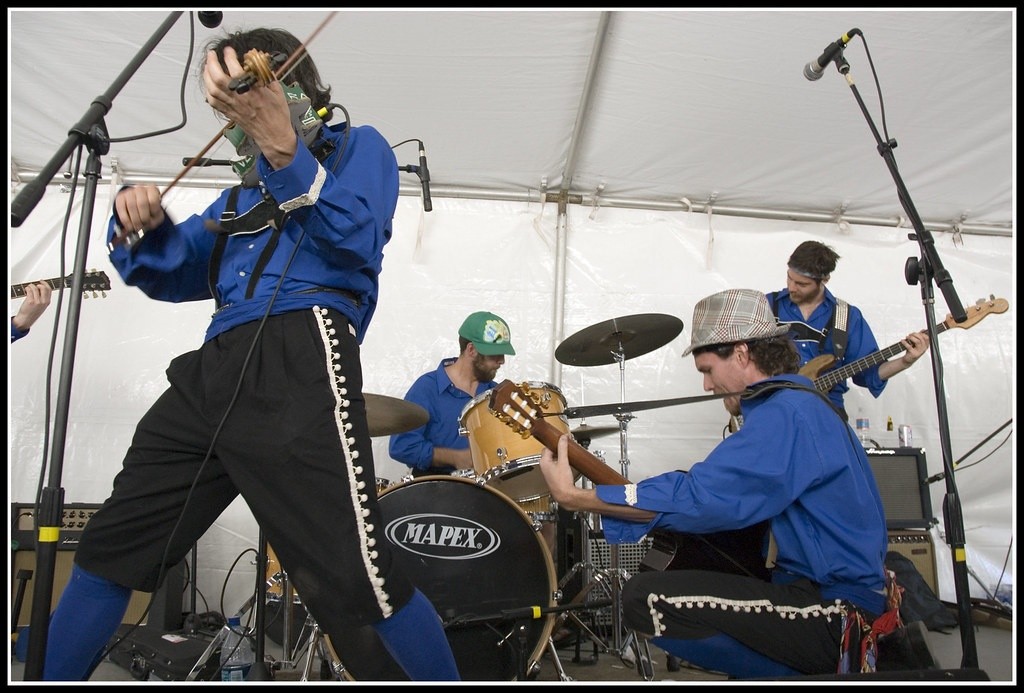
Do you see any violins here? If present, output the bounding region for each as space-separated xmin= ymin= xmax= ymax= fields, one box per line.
xmin=222 ymin=46 xmax=324 ymax=191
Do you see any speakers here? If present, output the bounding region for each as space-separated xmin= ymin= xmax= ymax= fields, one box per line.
xmin=884 ymin=528 xmax=940 ymax=601
xmin=862 ymin=447 xmax=934 ymax=530
xmin=11 ymin=503 xmax=187 ymax=664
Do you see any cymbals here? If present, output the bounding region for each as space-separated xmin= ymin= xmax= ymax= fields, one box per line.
xmin=554 ymin=312 xmax=684 ymax=367
xmin=362 ymin=391 xmax=432 ymax=439
xmin=570 ymin=425 xmax=622 ymax=440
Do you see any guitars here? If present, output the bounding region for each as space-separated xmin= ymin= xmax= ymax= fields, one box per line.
xmin=11 ymin=267 xmax=112 ymax=300
xmin=728 ymin=292 xmax=1010 ymax=439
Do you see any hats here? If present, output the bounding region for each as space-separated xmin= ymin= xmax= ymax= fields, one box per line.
xmin=458 ymin=311 xmax=516 ymax=356
xmin=681 ymin=289 xmax=791 ymax=357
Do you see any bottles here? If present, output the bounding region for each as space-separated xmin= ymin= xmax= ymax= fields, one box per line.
xmin=856 ymin=407 xmax=870 ymax=449
xmin=220 ymin=615 xmax=254 ymax=682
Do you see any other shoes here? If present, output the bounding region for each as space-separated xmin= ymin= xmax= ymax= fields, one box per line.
xmin=871 ymin=618 xmax=937 ymax=672
xmin=553 ymin=626 xmax=577 ymax=648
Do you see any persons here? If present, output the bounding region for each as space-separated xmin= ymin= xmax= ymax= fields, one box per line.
xmin=540 ymin=290 xmax=888 ymax=676
xmin=11 ymin=281 xmax=52 ymax=344
xmin=765 ymin=241 xmax=930 ymax=419
xmin=389 ymin=311 xmax=515 ymax=476
xmin=43 ymin=28 xmax=464 ymax=682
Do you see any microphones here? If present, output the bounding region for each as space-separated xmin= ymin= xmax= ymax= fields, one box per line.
xmin=803 ymin=28 xmax=857 ymax=82
xmin=198 ymin=11 xmax=223 ymax=28
xmin=418 ymin=142 xmax=433 ymax=212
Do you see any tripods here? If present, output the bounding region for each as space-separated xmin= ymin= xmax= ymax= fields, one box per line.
xmin=185 ymin=567 xmax=311 ymax=683
xmin=551 ymin=340 xmax=679 ymax=681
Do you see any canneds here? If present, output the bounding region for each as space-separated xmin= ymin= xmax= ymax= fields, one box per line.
xmin=898 ymin=424 xmax=914 ymax=449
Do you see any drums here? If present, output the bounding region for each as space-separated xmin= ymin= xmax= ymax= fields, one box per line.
xmin=314 ymin=471 xmax=560 ymax=682
xmin=266 ymin=475 xmax=389 ymax=606
xmin=459 ymin=380 xmax=586 ymax=504
xmin=451 ymin=467 xmax=558 ymax=525
